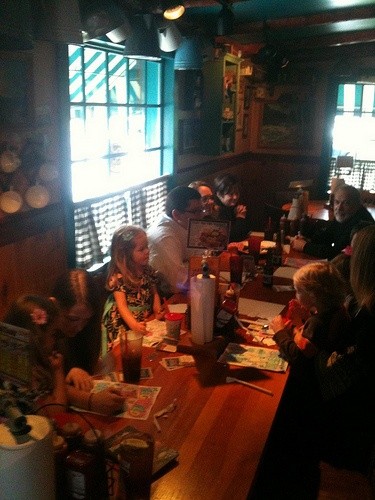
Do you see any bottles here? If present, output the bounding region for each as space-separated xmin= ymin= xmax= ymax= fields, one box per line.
xmin=49 ymin=420 xmax=108 ymax=500
xmin=216 ymin=283 xmax=238 ymax=339
xmin=280 ymin=213 xmax=309 ymax=253
xmin=272 ymin=232 xmax=284 ymax=266
xmin=262 ymin=247 xmax=274 ymax=286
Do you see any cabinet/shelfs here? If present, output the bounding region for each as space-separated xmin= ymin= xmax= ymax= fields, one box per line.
xmin=199 ymin=49 xmax=242 ymax=154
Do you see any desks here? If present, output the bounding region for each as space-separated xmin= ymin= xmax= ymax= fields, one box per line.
xmin=22 ymin=230 xmax=328 ymax=500
xmin=281 ymin=200 xmax=375 ymax=222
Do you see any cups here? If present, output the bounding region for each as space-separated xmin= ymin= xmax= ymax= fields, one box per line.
xmin=248 ymin=235 xmax=261 ymax=266
xmin=117 ymin=433 xmax=155 ymax=500
xmin=230 ymin=283 xmax=241 ymax=302
xmin=119 ymin=331 xmax=145 ymax=383
xmin=228 ymin=254 xmax=244 ymax=285
xmin=164 ymin=313 xmax=183 ymax=340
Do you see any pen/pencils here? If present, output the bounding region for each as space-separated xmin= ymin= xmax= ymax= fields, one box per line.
xmin=230 ymin=377 xmax=276 ymax=395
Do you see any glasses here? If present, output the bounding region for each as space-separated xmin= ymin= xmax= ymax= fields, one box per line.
xmin=182 ymin=208 xmax=204 ymax=214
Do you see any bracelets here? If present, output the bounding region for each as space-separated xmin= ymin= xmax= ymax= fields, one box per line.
xmin=88 ymin=393 xmax=94 ymax=411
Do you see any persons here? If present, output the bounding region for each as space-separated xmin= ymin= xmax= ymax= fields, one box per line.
xmin=146 ymin=175 xmax=248 ymax=299
xmin=0 ymin=268 xmax=126 ymax=417
xmin=262 ymin=186 xmax=375 ymax=500
xmin=104 ymin=227 xmax=167 ymax=354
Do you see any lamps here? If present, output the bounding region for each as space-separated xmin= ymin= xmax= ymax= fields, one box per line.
xmin=211 ymin=5 xmax=235 ymax=42
xmin=251 ymin=45 xmax=283 ymax=74
xmin=0 ymin=0 xmax=203 ymax=71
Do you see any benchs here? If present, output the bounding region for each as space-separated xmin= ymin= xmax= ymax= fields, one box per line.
xmin=312 ymin=460 xmax=375 ymax=500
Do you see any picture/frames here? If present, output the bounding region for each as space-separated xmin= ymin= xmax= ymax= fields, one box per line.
xmin=243 ymin=114 xmax=248 ymax=138
xmin=243 ymin=86 xmax=251 ymax=110
xmin=179 ymin=119 xmax=197 ymax=155
